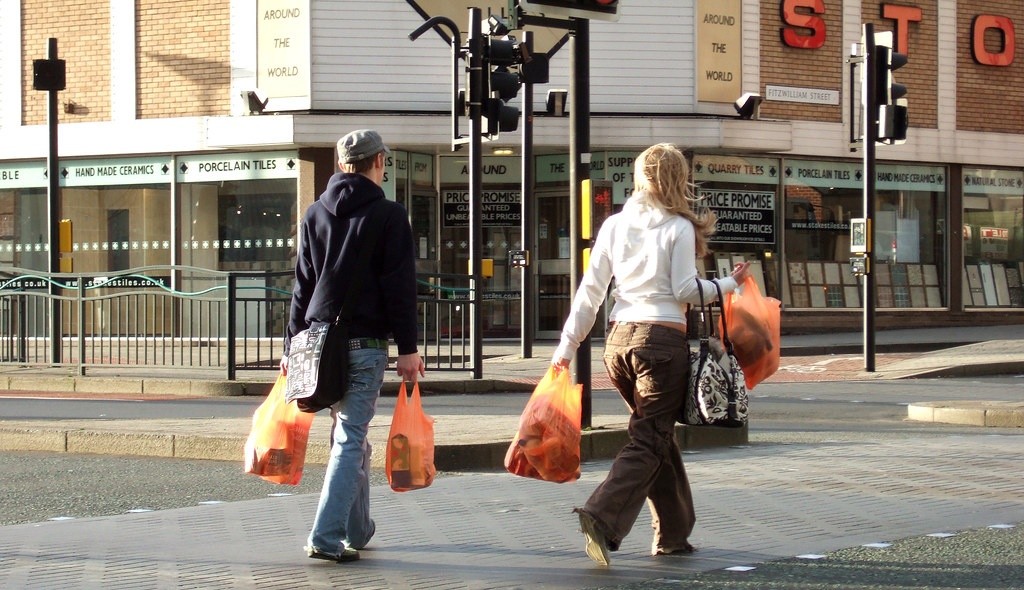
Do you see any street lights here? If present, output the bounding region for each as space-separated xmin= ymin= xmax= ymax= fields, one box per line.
xmin=518 ymin=51 xmax=550 ymax=359
xmin=31 ymin=57 xmax=67 ymax=366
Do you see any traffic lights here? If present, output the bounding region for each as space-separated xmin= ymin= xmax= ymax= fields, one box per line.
xmin=466 ymin=37 xmax=522 ymax=139
xmin=509 ymin=251 xmax=529 ymax=267
xmin=848 ymin=218 xmax=873 ymax=254
xmin=858 ymin=32 xmax=908 ymax=143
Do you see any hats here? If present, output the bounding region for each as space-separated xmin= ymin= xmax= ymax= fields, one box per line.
xmin=337 ymin=129 xmax=391 ymax=163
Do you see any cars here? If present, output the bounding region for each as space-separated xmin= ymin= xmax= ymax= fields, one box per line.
xmin=876 ymin=230 xmax=923 ymax=264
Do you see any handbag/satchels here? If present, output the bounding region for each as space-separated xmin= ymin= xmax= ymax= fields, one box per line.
xmin=244 ymin=370 xmax=314 ymax=486
xmin=504 ymin=366 xmax=582 ymax=484
xmin=285 ymin=323 xmax=350 ymax=413
xmin=678 ymin=278 xmax=748 ymax=428
xmin=718 ymin=274 xmax=781 ymax=390
xmin=385 ymin=381 xmax=436 ymax=493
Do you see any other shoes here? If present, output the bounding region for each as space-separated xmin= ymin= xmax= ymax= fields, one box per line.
xmin=578 ymin=510 xmax=610 ymax=566
xmin=352 ymin=517 xmax=375 ymax=549
xmin=651 ymin=541 xmax=697 ymax=557
xmin=308 ymin=547 xmax=360 ymax=561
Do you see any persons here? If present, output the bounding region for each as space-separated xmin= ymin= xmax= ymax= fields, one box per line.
xmin=555 ymin=143 xmax=753 ymax=568
xmin=278 ymin=129 xmax=426 ymax=563
